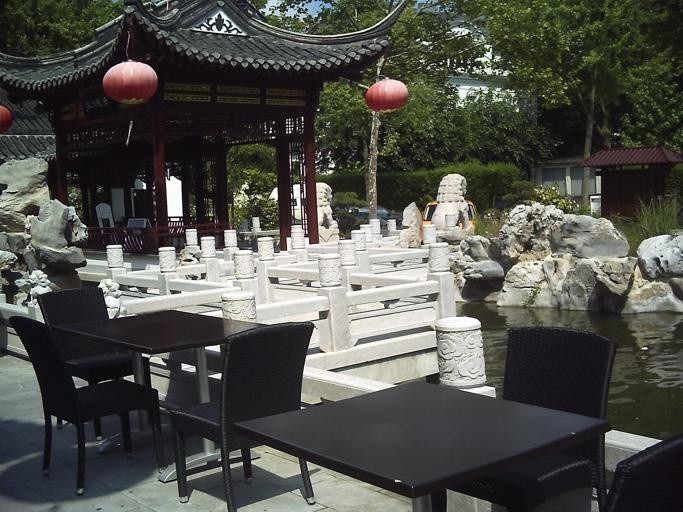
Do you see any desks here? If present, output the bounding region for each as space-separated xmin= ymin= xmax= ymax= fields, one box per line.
xmin=52 ymin=309 xmax=271 ymax=482
xmin=231 ymin=379 xmax=611 ymax=512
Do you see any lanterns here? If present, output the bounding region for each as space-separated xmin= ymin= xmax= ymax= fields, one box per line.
xmin=101 ymin=58 xmax=159 ymax=110
xmin=0 ymin=105 xmax=13 ymax=135
xmin=365 ymin=75 xmax=410 ymax=115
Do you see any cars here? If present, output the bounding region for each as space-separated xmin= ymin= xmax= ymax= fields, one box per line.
xmin=334 ymin=204 xmax=402 ymax=230
xmin=423 ymin=198 xmax=479 ymax=235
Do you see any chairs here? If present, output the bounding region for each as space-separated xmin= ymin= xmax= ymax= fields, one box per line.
xmin=9 ymin=315 xmax=165 ymax=494
xmin=533 ymin=435 xmax=681 ymax=509
xmin=35 ymin=286 xmax=153 ymax=440
xmin=445 ymin=326 xmax=615 ymax=511
xmin=438 ymin=325 xmax=683 ymax=511
xmin=170 ymin=321 xmax=314 ymax=511
